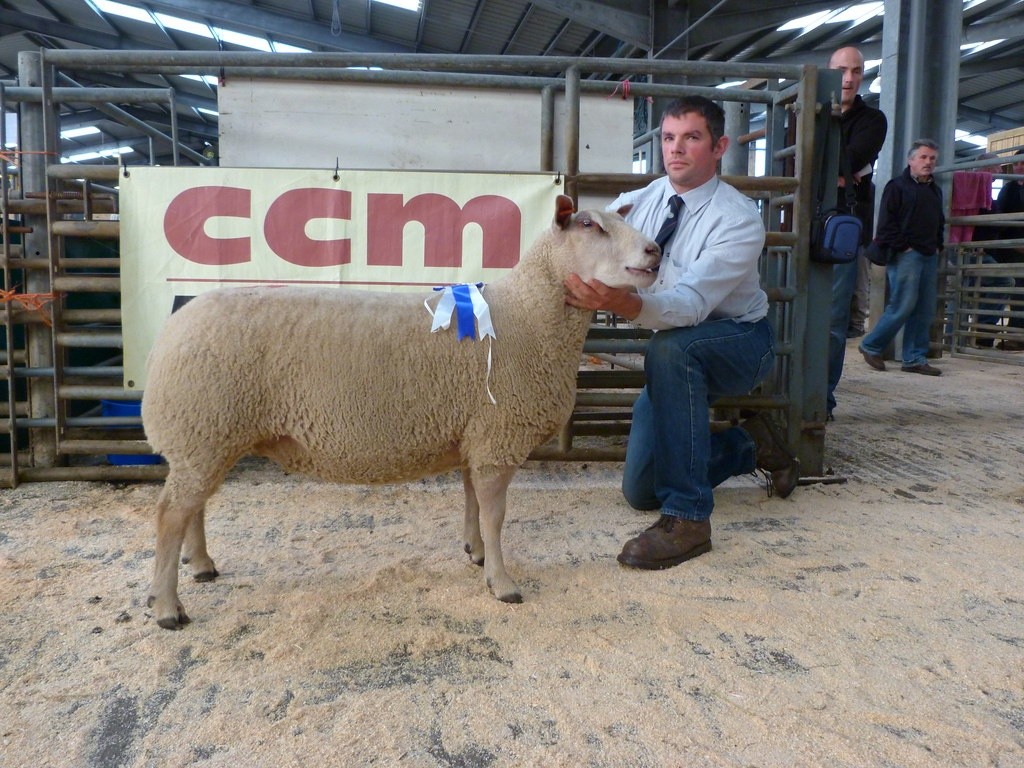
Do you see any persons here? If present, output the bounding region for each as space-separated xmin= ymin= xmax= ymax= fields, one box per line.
xmin=858 ymin=138 xmax=946 ymax=375
xmin=971 ymin=148 xmax=1024 ymax=351
xmin=564 ymin=94 xmax=800 ymax=572
xmin=826 ymin=46 xmax=888 ymax=421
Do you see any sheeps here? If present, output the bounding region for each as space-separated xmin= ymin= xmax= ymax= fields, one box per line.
xmin=142 ymin=195 xmax=662 ymax=632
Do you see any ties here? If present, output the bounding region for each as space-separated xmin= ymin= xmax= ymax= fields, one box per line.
xmin=652 ymin=195 xmax=684 ymax=272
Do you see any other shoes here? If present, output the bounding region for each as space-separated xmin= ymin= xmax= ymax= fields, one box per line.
xmin=617 ymin=515 xmax=713 ymax=570
xmin=858 ymin=345 xmax=885 ymax=370
xmin=901 ymin=363 xmax=942 ymax=376
xmin=995 ymin=340 xmax=1024 ymax=350
xmin=825 ymin=409 xmax=835 ymax=426
xmin=740 ymin=412 xmax=799 ymax=499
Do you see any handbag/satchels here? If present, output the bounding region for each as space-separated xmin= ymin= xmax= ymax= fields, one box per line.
xmin=864 ymin=239 xmax=893 ymax=266
xmin=819 ymin=208 xmax=863 ymax=264
